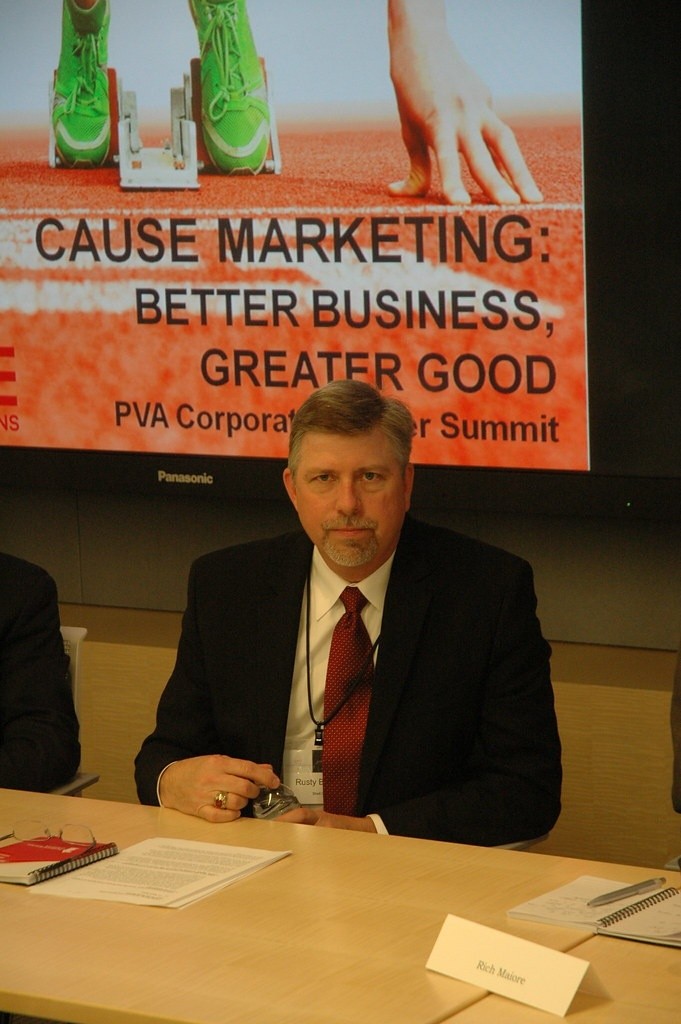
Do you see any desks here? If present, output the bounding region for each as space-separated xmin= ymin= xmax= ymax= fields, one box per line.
xmin=0 ymin=787 xmax=681 ymax=1024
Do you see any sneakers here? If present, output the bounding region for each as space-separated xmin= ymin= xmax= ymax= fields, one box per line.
xmin=190 ymin=0 xmax=270 ymax=177
xmin=52 ymin=0 xmax=113 ymax=170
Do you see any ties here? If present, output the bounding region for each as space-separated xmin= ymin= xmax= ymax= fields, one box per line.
xmin=324 ymin=586 xmax=375 ymax=815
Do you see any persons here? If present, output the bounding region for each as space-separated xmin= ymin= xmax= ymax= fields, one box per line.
xmin=0 ymin=553 xmax=83 ymax=792
xmin=134 ymin=380 xmax=563 ymax=848
xmin=50 ymin=0 xmax=543 ymax=205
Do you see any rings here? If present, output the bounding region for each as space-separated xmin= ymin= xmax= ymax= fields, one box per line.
xmin=215 ymin=790 xmax=230 ymax=811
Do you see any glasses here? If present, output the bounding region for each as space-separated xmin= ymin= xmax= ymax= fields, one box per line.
xmin=0 ymin=820 xmax=98 ymax=874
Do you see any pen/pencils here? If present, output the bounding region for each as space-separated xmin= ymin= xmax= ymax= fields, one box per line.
xmin=587 ymin=877 xmax=666 ymax=908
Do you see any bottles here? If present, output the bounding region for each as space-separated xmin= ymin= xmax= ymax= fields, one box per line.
xmin=252 ymin=763 xmax=304 ymax=820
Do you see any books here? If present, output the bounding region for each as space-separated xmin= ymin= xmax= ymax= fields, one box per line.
xmin=1 ymin=835 xmax=118 ymax=887
xmin=506 ymin=873 xmax=681 ymax=948
